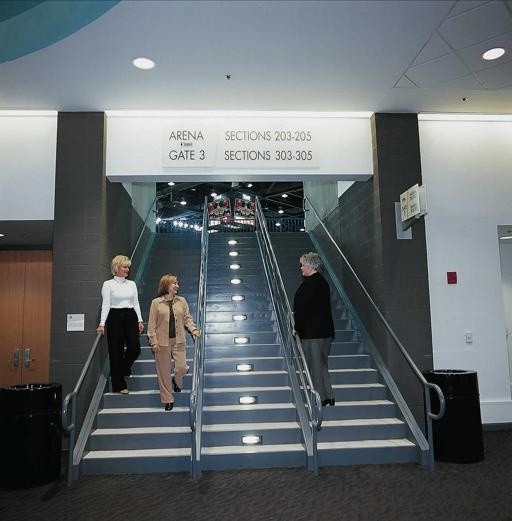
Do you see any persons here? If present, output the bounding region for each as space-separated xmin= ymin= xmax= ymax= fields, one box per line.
xmin=97 ymin=254 xmax=144 ymax=394
xmin=147 ymin=274 xmax=201 ymax=410
xmin=292 ymin=252 xmax=335 ymax=407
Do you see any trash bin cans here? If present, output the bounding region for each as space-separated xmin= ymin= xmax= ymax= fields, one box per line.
xmin=423 ymin=369 xmax=484 ymax=463
xmin=8 ymin=383 xmax=63 ymax=490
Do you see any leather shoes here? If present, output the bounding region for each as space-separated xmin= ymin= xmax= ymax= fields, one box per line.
xmin=315 ymin=399 xmax=328 ymax=406
xmin=164 ymin=402 xmax=173 ymax=410
xmin=325 ymin=398 xmax=335 ymax=406
xmin=120 ymin=389 xmax=129 ymax=394
xmin=172 ymin=376 xmax=182 ymax=392
